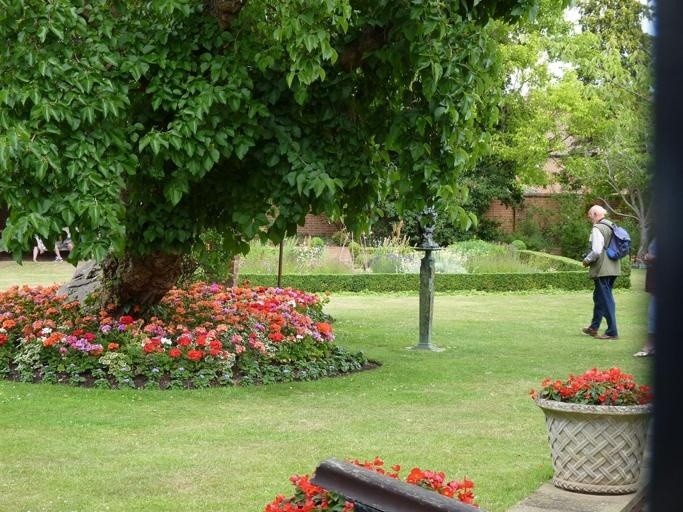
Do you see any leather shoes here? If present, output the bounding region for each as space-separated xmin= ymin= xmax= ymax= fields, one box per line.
xmin=595 ymin=333 xmax=618 ymax=340
xmin=581 ymin=325 xmax=597 ymax=337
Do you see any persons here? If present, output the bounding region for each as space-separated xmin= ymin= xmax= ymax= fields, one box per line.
xmin=581 ymin=205 xmax=622 ymax=341
xmin=54 ymin=230 xmax=72 ymax=261
xmin=30 ymin=235 xmax=45 ymax=263
xmin=632 ymin=239 xmax=656 ymax=358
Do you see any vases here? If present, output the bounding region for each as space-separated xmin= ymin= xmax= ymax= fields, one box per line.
xmin=538 ymin=399 xmax=653 ymax=495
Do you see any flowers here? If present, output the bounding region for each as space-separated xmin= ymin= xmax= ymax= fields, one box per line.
xmin=528 ymin=366 xmax=654 ymax=406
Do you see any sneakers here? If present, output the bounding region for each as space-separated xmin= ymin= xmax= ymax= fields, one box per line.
xmin=631 ymin=349 xmax=655 ymax=358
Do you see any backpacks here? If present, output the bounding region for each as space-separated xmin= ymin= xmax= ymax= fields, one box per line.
xmin=595 ymin=221 xmax=631 ymax=261
